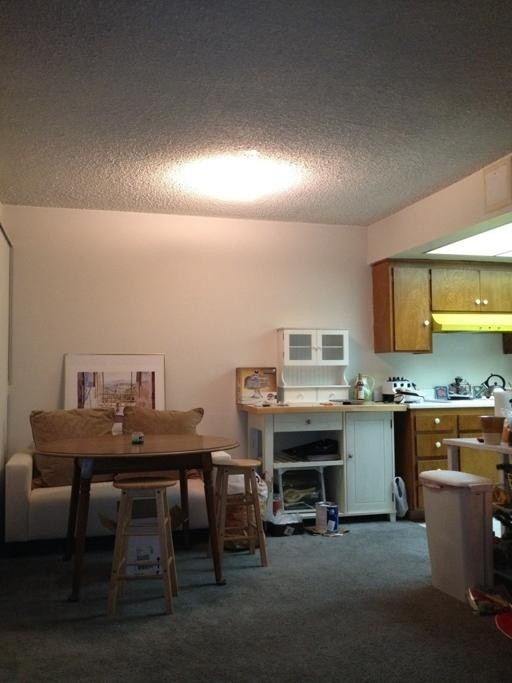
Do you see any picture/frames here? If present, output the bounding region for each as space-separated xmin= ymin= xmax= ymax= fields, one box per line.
xmin=63 ymin=352 xmax=166 ymax=411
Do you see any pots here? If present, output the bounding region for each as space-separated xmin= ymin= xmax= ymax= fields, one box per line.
xmin=448 ymin=376 xmax=471 ymax=394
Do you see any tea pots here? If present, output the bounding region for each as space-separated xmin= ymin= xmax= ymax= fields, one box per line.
xmin=482 ymin=372 xmax=506 ymax=398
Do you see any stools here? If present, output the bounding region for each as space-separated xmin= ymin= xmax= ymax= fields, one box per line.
xmin=105 ymin=478 xmax=178 ymax=616
xmin=212 ymin=458 xmax=267 ymax=570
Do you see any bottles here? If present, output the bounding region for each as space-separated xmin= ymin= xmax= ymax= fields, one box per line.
xmin=356 ymin=373 xmax=363 ymax=400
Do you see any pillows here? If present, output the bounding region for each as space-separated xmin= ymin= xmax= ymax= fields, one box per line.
xmin=30 ymin=407 xmax=119 ymax=490
xmin=122 ymin=406 xmax=205 ymax=482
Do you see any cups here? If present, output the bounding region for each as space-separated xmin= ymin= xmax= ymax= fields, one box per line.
xmin=479 ymin=416 xmax=506 ymax=445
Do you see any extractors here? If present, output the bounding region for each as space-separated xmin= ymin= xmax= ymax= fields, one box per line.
xmin=431 ymin=312 xmax=512 ymax=333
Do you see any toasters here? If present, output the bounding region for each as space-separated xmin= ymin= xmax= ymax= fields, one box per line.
xmin=383 ymin=376 xmax=425 ymax=402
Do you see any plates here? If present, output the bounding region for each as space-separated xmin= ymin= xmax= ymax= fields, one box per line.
xmin=351 ymin=399 xmax=365 ymax=405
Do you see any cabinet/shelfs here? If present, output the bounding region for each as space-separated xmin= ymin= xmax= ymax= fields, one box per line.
xmin=246 ymin=411 xmax=347 ymax=523
xmin=346 ymin=412 xmax=397 ymax=524
xmin=411 ymin=412 xmax=458 ymax=513
xmin=277 ymin=327 xmax=351 ymax=406
xmin=372 ymin=262 xmax=431 ymax=353
xmin=458 ymin=412 xmax=502 ymax=483
xmin=430 ymin=266 xmax=512 ymax=313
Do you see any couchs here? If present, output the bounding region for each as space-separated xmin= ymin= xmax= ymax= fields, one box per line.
xmin=5 ymin=442 xmax=267 ymax=544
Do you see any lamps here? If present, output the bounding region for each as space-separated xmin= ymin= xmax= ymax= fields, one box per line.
xmin=161 ymin=149 xmax=307 ymax=206
